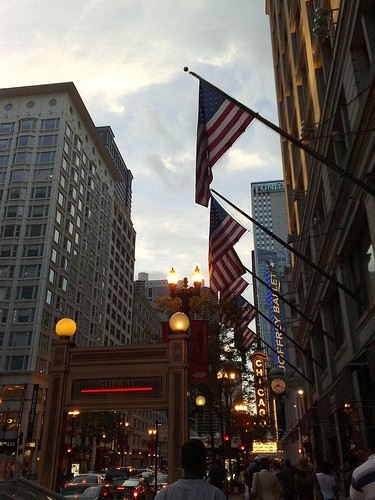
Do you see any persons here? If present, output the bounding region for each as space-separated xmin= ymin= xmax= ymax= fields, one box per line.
xmin=154 ymin=439 xmax=226 ymax=500
xmin=209 ymin=460 xmax=225 ymax=491
xmin=239 ymin=455 xmax=337 ymax=500
xmin=349 ymin=453 xmax=375 ymax=500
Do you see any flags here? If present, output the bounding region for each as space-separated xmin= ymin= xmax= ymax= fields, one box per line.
xmin=234 ymin=329 xmax=256 ymax=353
xmin=209 ymin=195 xmax=246 ymax=294
xmin=196 ymin=80 xmax=254 ymax=207
xmin=218 ymin=277 xmax=259 ymax=331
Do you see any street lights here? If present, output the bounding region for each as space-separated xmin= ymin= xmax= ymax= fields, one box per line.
xmin=165 ymin=267 xmax=204 ymax=363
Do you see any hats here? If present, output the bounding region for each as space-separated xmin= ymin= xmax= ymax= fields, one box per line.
xmin=291 ymin=456 xmax=314 ymax=472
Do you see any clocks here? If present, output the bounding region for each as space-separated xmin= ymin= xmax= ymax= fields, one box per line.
xmin=271 ymin=378 xmax=285 ymax=393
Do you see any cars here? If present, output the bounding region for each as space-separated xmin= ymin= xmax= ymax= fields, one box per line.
xmin=65 ymin=465 xmax=168 ymax=500
